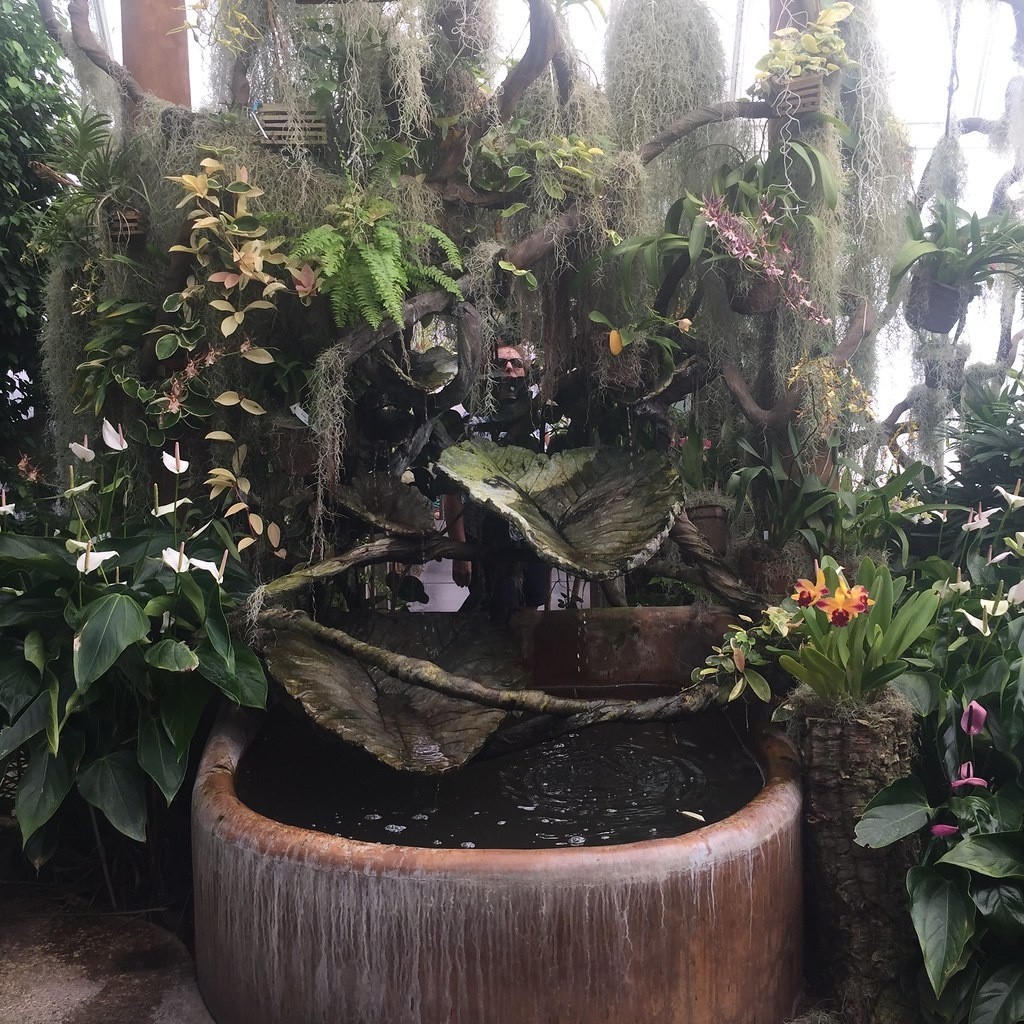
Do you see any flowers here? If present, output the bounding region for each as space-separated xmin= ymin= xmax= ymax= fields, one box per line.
xmin=941 ymin=471 xmax=1024 ymax=679
xmin=0 ymin=421 xmax=271 ymax=848
xmin=768 ymin=549 xmax=940 ymax=699
xmin=860 ymin=685 xmax=1024 ymax=1024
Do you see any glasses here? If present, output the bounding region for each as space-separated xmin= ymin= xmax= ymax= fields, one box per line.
xmin=495 ymin=358 xmax=526 ymax=369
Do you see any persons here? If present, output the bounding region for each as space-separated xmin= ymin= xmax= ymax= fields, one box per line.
xmin=442 ymin=347 xmax=564 ymax=615
